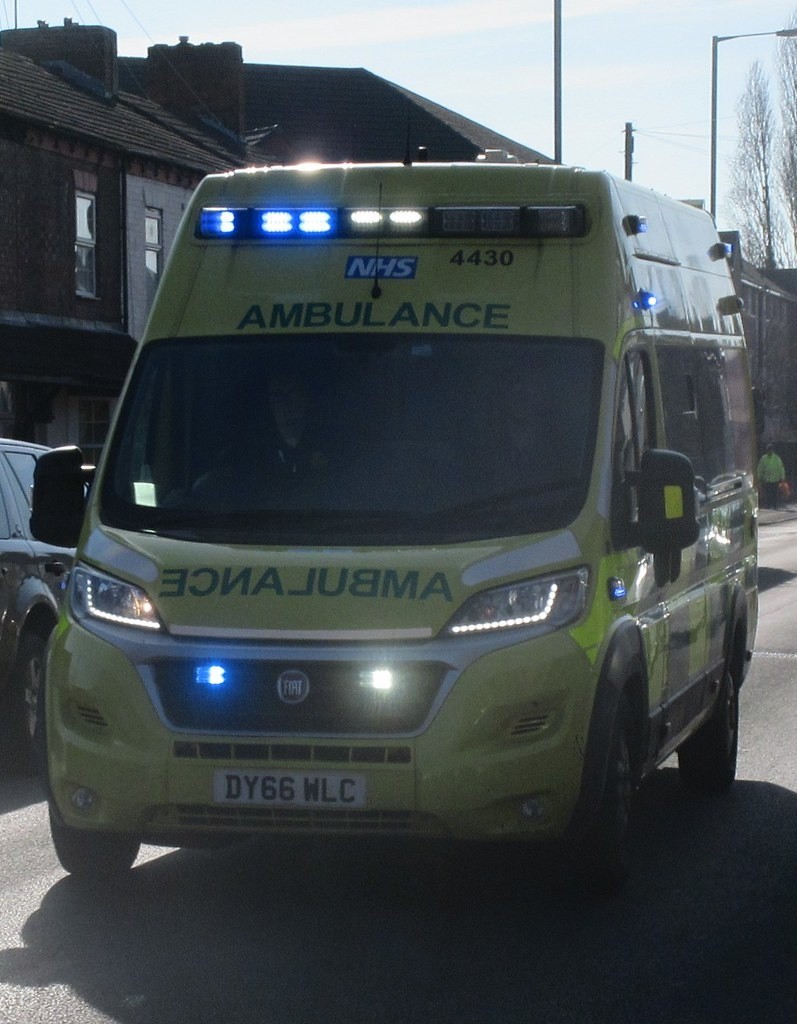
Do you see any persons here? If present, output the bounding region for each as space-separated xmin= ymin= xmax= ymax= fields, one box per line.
xmin=757 ymin=444 xmax=786 ymax=507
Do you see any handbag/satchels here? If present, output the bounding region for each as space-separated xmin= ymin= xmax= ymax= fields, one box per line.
xmin=778 ymin=482 xmax=790 ymax=501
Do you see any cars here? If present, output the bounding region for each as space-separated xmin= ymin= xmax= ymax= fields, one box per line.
xmin=0 ymin=438 xmax=78 ymax=767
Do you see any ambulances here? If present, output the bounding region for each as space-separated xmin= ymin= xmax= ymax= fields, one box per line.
xmin=46 ymin=149 xmax=758 ymax=919
